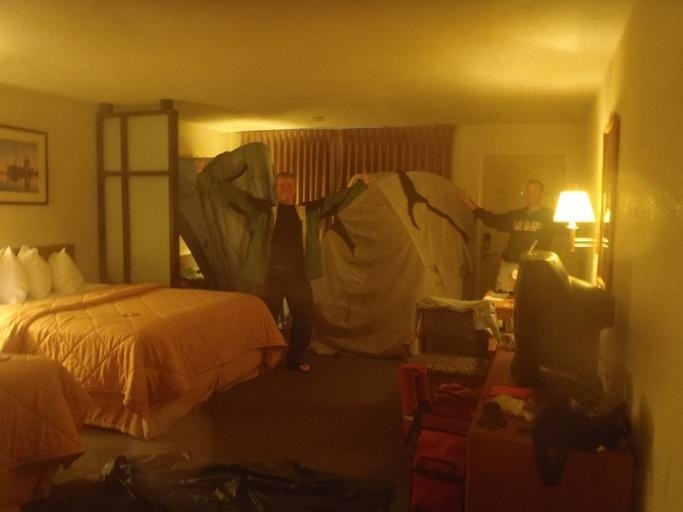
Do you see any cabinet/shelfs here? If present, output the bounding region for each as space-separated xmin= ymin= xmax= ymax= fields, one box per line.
xmin=463 ymin=350 xmax=638 ymax=512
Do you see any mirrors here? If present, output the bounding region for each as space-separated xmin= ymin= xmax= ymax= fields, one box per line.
xmin=595 ymin=112 xmax=620 ymax=291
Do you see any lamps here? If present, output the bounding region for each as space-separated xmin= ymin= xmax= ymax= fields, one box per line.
xmin=552 ymin=189 xmax=599 ymax=253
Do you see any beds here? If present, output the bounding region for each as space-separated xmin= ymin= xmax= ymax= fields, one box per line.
xmin=0 ymin=242 xmax=288 ymax=441
xmin=0 ymin=352 xmax=92 ymax=512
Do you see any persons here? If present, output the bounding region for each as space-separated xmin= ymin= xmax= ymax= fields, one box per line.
xmin=207 ymin=154 xmax=371 ymax=377
xmin=456 ymin=177 xmax=570 ymax=296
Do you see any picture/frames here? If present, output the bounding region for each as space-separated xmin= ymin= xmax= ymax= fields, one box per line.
xmin=0 ymin=124 xmax=49 ymax=206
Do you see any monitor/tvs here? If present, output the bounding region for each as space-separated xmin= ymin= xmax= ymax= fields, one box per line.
xmin=514 ymin=250 xmax=616 ymax=389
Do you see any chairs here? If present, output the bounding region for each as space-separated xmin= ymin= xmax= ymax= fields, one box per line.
xmin=410 ymin=299 xmax=494 ymax=384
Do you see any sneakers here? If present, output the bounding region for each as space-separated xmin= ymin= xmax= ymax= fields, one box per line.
xmin=300 ymin=362 xmax=310 ymax=372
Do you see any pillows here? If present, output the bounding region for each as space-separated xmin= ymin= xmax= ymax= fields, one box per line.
xmin=0 ymin=244 xmax=84 ymax=307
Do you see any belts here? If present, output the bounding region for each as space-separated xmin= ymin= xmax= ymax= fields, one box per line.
xmin=270 ymin=265 xmax=298 ymax=272
